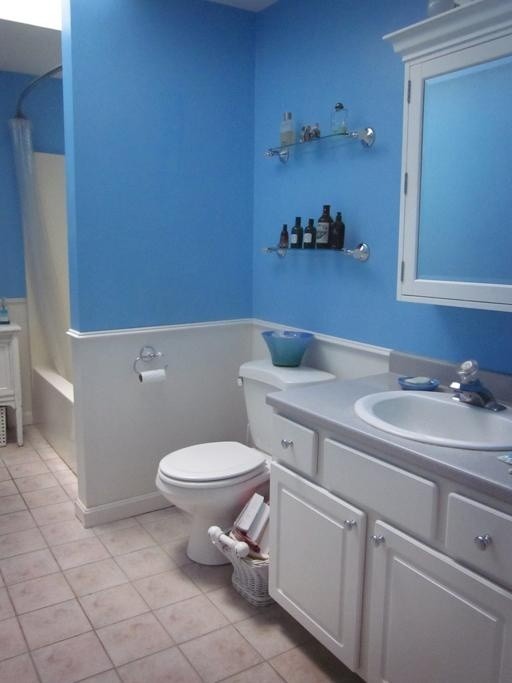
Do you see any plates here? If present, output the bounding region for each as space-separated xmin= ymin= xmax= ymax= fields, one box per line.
xmin=398 ymin=374 xmax=442 ymax=390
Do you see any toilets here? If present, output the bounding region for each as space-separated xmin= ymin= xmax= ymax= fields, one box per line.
xmin=156 ymin=360 xmax=336 ymax=565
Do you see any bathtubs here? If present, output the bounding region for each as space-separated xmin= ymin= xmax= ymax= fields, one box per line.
xmin=32 ymin=364 xmax=77 ymax=478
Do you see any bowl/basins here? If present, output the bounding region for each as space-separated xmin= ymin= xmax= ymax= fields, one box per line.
xmin=261 ymin=330 xmax=313 ymax=367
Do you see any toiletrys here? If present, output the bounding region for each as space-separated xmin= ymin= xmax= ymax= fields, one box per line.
xmin=331 ymin=101 xmax=349 ymax=136
xmin=278 ymin=223 xmax=291 ymax=247
xmin=278 ymin=111 xmax=296 ymax=146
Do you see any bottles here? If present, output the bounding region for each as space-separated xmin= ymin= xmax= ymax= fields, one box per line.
xmin=299 ymin=122 xmax=321 ymax=142
xmin=0 ymin=295 xmax=10 ymax=325
xmin=279 ymin=224 xmax=288 ymax=248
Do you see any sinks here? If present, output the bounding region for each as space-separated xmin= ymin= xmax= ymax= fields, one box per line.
xmin=354 ymin=389 xmax=512 ymax=453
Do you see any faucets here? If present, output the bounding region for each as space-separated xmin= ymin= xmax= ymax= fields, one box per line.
xmin=449 ymin=359 xmax=507 ymax=413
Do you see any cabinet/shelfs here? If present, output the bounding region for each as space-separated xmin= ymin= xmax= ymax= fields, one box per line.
xmin=0 ymin=318 xmax=25 ymax=448
xmin=252 ymin=410 xmax=512 ymax=682
xmin=381 ymin=0 xmax=511 ymax=313
xmin=262 ymin=126 xmax=375 ymax=262
